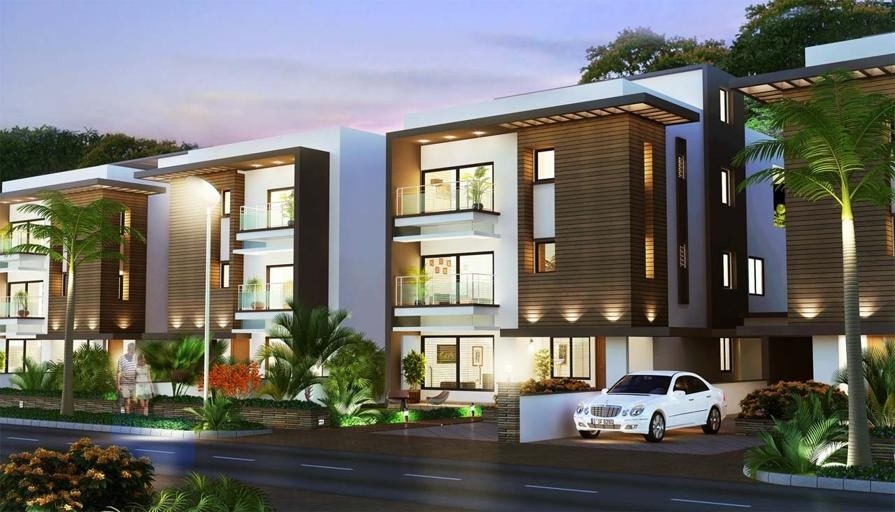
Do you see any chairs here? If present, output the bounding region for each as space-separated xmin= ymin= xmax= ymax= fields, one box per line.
xmin=425 ymin=390 xmax=450 ymax=405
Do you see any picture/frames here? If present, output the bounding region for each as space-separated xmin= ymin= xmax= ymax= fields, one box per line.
xmin=472 ymin=345 xmax=484 ymax=367
xmin=437 ymin=344 xmax=457 ymax=364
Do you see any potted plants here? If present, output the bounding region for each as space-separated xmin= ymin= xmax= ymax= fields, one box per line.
xmin=279 ymin=189 xmax=295 ymax=226
xmin=400 ymin=350 xmax=426 ymax=404
xmin=244 ymin=278 xmax=265 ymax=310
xmin=404 ymin=264 xmax=432 ymax=306
xmin=14 ymin=289 xmax=30 ymax=317
xmin=463 ymin=165 xmax=493 ymax=210
